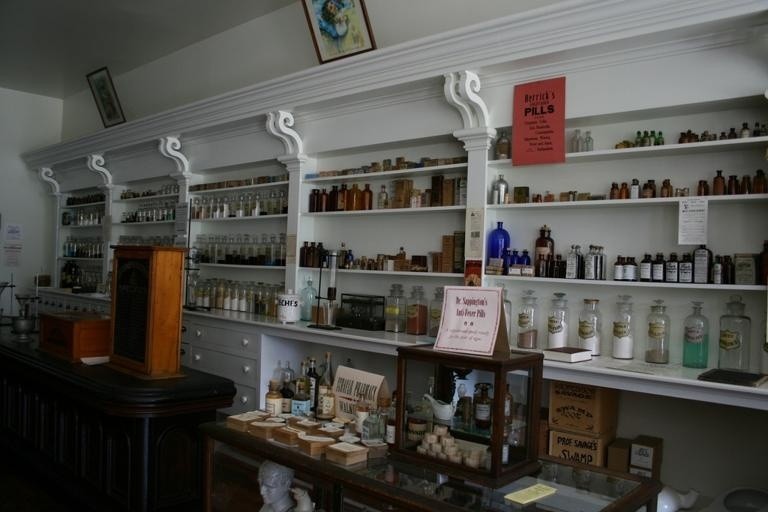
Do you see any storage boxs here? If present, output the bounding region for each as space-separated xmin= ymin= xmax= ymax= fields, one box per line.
xmin=509 ymin=375 xmax=665 ymax=486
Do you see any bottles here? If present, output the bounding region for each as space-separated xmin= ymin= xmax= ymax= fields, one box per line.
xmin=486 ymin=217 xmax=734 ymax=287
xmin=265 ymin=348 xmax=334 ymax=418
xmin=568 ymin=190 xmax=583 ymax=200
xmin=120 ymin=182 xmax=179 ymax=244
xmin=354 ymin=388 xmax=426 ymax=446
xmin=410 ymin=188 xmax=431 ymax=210
xmin=191 ymin=231 xmax=286 ymax=265
xmin=474 ymin=382 xmax=514 ymax=429
xmin=506 ymin=292 xmax=767 ymax=369
xmin=758 ymin=238 xmax=768 ymax=284
xmin=494 ymin=128 xmax=511 ymax=160
xmin=491 ymin=172 xmax=508 ymax=205
xmin=61 ymin=192 xmax=106 ymax=285
xmin=299 ymin=241 xmax=407 ymax=272
xmin=191 ymin=189 xmax=287 ymax=219
xmin=385 ymin=282 xmax=445 ymax=335
xmin=608 ymin=167 xmax=766 ymax=198
xmin=309 ymin=181 xmax=388 ymax=210
xmin=571 ymin=128 xmax=593 ymax=154
xmin=500 ymin=430 xmax=512 ymax=467
xmin=187 ymin=275 xmax=315 ymax=324
xmin=634 ymin=119 xmax=767 ymax=148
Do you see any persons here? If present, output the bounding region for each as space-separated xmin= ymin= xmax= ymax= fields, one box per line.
xmin=322 ymin=1 xmax=349 ymax=37
xmin=256 ymin=460 xmax=316 ymax=512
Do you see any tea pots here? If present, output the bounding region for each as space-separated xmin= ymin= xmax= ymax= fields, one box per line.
xmin=423 ymin=392 xmax=457 ymax=421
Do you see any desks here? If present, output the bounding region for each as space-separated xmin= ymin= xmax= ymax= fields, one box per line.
xmin=2 ymin=325 xmax=234 ymax=512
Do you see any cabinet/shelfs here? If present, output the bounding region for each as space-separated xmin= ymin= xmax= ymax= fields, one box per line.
xmin=55 ymin=186 xmax=107 ymax=291
xmin=185 ymin=161 xmax=288 ymax=318
xmin=199 ymin=407 xmax=656 ymax=512
xmin=294 ymin=132 xmax=466 ymax=335
xmin=386 ymin=331 xmax=544 ymax=490
xmin=107 ymin=171 xmax=179 ymax=291
xmin=483 ymin=91 xmax=768 ymax=415
xmin=32 ymin=287 xmax=311 ymax=416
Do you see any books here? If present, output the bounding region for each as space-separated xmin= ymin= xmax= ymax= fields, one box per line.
xmin=698 ymin=369 xmax=768 ymax=387
xmin=541 ymin=347 xmax=592 ymax=363
